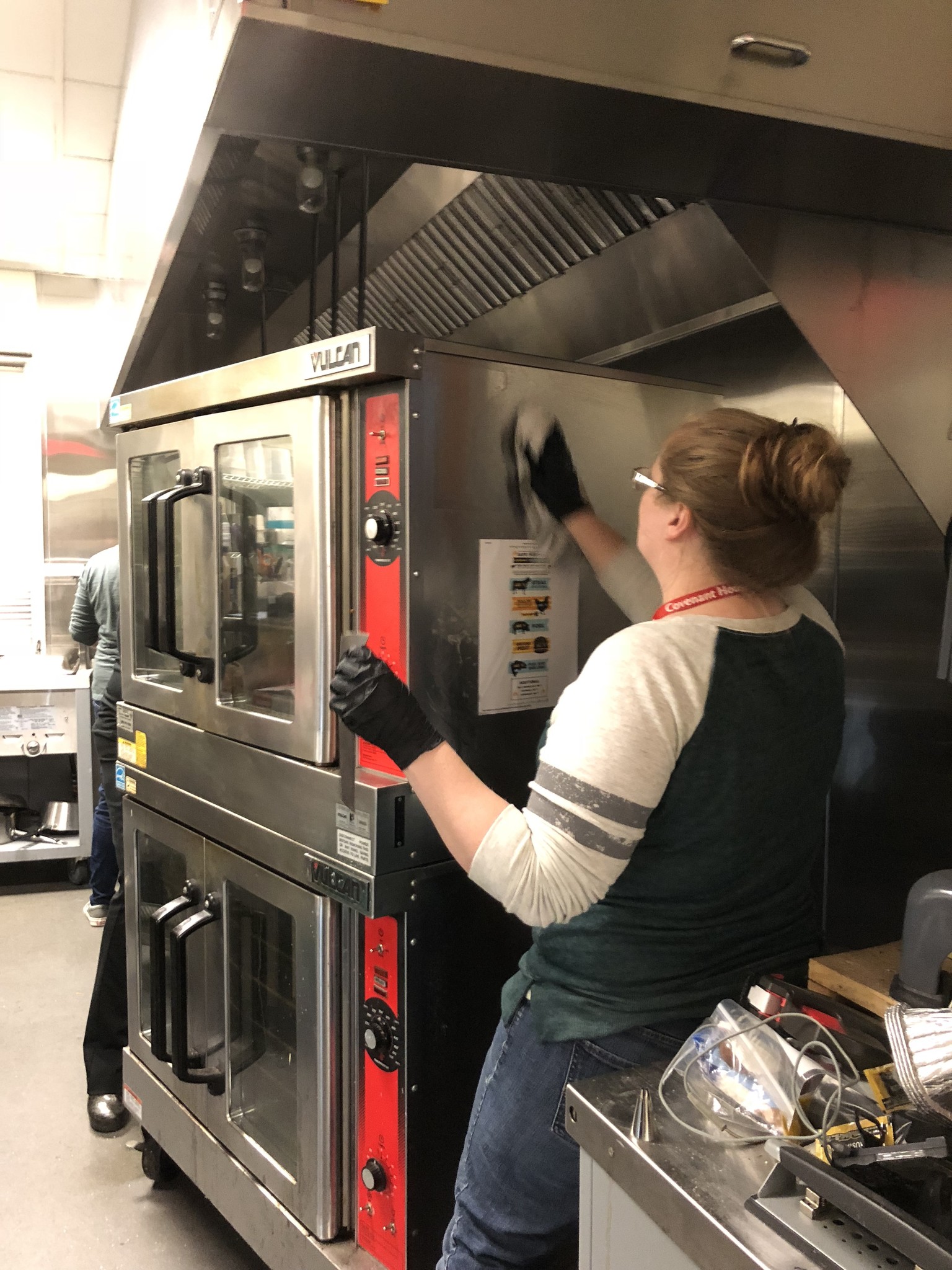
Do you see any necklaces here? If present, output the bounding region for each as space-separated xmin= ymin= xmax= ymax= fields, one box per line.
xmin=651 ymin=576 xmax=750 ymax=618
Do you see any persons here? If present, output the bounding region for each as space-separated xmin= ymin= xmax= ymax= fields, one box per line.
xmin=68 ymin=524 xmax=124 ymax=1133
xmin=330 ymin=407 xmax=855 ymax=1269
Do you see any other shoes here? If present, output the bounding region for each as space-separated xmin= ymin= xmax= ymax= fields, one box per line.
xmin=83 ymin=899 xmax=108 ymax=925
xmin=88 ymin=1091 xmax=130 ymax=1133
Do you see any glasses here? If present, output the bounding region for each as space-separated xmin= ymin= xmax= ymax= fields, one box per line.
xmin=630 ymin=466 xmax=673 ymax=495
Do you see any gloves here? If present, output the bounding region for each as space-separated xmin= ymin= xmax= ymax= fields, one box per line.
xmin=328 ymin=643 xmax=446 ymax=772
xmin=524 ymin=416 xmax=586 ymax=522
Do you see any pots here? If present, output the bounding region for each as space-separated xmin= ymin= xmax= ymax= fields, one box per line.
xmin=10 ymin=801 xmax=79 ymax=841
xmin=0 ymin=810 xmax=67 ymax=845
xmin=0 ymin=794 xmax=30 ymax=809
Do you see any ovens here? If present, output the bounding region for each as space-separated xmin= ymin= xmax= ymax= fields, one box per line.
xmin=110 ymin=322 xmax=727 ymax=1270
